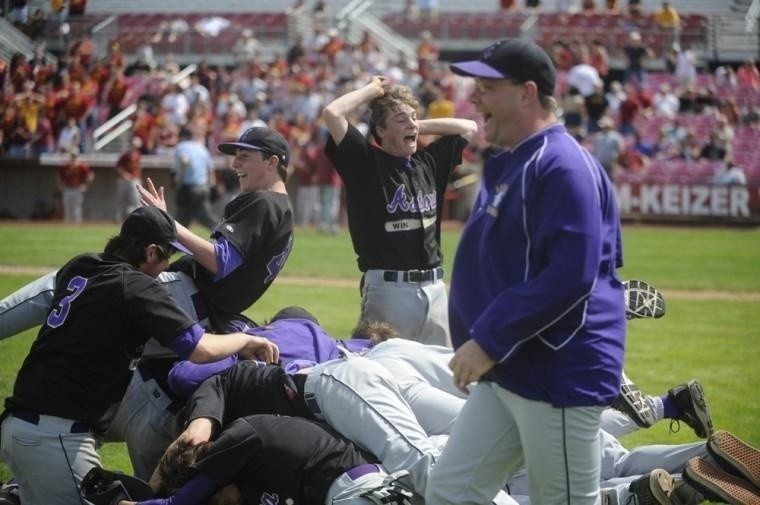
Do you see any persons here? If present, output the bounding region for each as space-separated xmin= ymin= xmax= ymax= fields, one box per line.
xmin=323 ymin=76 xmax=477 ymax=346
xmin=81 ymin=283 xmax=759 ymax=505
xmin=422 ymin=38 xmax=627 ymax=505
xmin=1 ymin=0 xmax=760 ymax=235
xmin=0 ymin=205 xmax=279 ymax=505
xmin=0 ymin=127 xmax=295 ymax=349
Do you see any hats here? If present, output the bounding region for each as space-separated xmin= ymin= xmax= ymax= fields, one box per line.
xmin=119 ymin=203 xmax=193 ymax=258
xmin=446 ymin=36 xmax=559 ymax=98
xmin=216 ymin=123 xmax=287 ymax=169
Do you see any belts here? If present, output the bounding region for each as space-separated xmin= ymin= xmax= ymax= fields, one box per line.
xmin=344 ymin=463 xmax=392 ymax=481
xmin=383 ymin=266 xmax=443 ymax=283
xmin=2 ymin=395 xmax=95 ymax=437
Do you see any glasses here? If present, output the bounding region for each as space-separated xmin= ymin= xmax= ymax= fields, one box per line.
xmin=473 ymin=80 xmax=522 ymax=93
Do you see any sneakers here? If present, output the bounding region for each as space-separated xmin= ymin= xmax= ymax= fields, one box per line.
xmin=703 ymin=429 xmax=760 ymax=488
xmin=628 ymin=467 xmax=682 ymax=504
xmin=662 ymin=381 xmax=715 ymax=442
xmin=620 ymin=278 xmax=666 ymax=322
xmin=608 ymin=367 xmax=652 ymax=431
xmin=683 ymin=455 xmax=760 ymax=504
